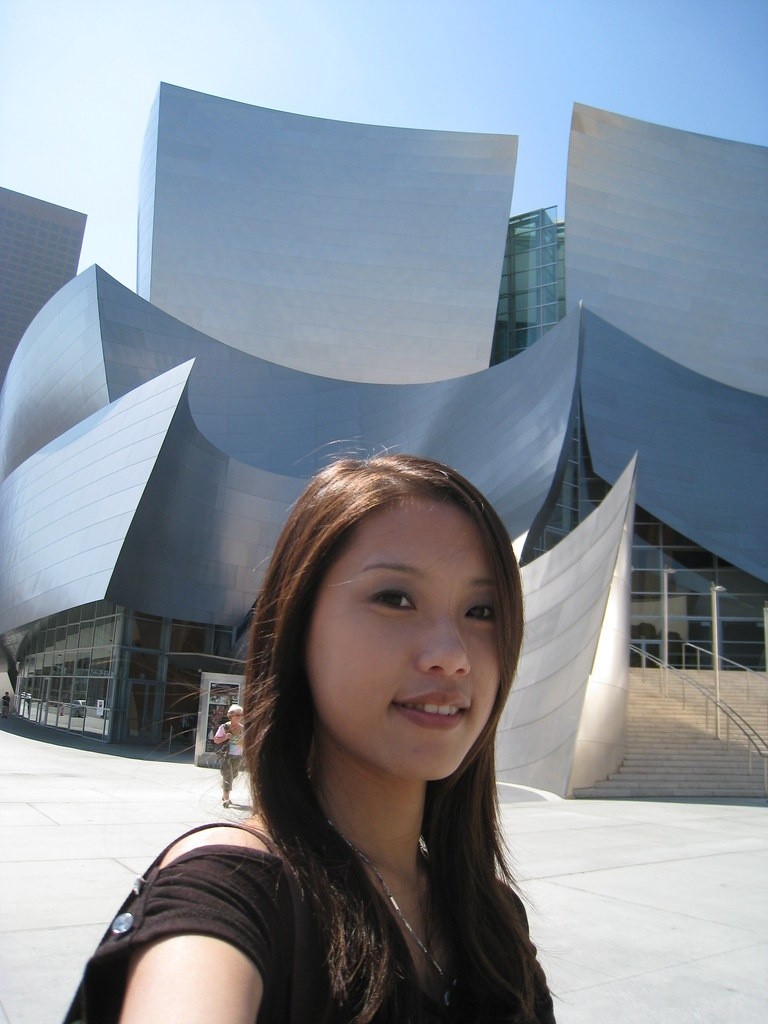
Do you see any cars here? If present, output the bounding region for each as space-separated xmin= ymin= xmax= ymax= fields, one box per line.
xmin=72 ymin=699 xmax=85 ymax=717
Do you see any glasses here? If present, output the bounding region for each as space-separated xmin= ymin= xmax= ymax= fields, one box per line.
xmin=232 ymin=713 xmax=243 ymax=716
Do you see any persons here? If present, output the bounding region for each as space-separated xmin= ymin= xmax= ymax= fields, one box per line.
xmin=214 ymin=705 xmax=245 ymax=807
xmin=181 ymin=708 xmax=194 ymax=745
xmin=67 ymin=452 xmax=556 ymax=1024
xmin=2 ymin=692 xmax=10 ymax=718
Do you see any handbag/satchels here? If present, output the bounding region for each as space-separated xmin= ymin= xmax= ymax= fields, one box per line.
xmin=215 ymin=723 xmax=229 ymax=763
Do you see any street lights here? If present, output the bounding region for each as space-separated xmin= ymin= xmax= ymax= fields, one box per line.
xmin=662 ymin=567 xmax=678 ymax=697
xmin=710 ymin=585 xmax=728 ymax=740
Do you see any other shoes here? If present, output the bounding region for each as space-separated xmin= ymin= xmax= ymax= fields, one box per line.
xmin=222 ymin=797 xmax=229 ymax=808
xmin=229 ymin=799 xmax=232 ymax=804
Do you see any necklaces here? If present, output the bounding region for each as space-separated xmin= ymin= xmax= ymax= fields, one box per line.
xmin=325 ymin=812 xmax=460 ymax=1010
